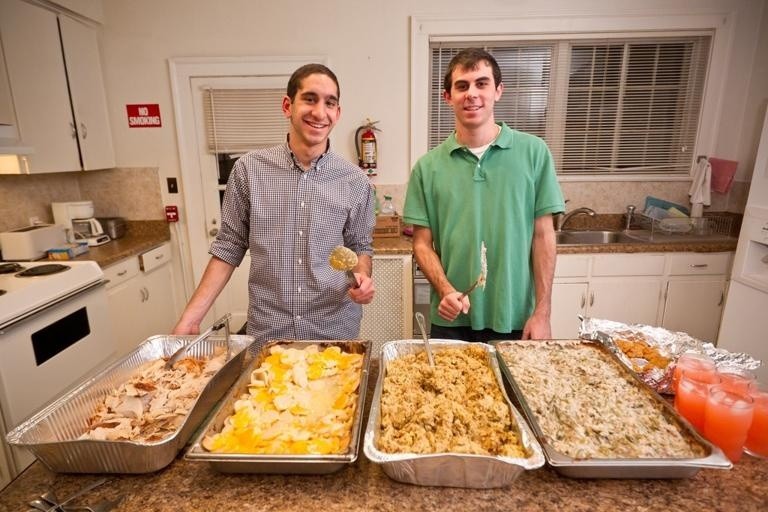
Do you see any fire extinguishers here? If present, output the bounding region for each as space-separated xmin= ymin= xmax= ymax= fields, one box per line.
xmin=353 ymin=118 xmax=383 ymax=176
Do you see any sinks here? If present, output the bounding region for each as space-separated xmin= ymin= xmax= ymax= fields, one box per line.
xmin=555 ymin=231 xmax=578 ymax=244
xmin=566 ymin=230 xmax=646 ymax=244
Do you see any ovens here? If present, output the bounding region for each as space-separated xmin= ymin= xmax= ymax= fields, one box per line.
xmin=0 ymin=279 xmax=117 ymax=472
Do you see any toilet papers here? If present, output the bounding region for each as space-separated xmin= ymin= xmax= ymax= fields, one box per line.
xmin=690 ymin=203 xmax=703 ymax=225
xmin=667 ymin=207 xmax=688 ymax=218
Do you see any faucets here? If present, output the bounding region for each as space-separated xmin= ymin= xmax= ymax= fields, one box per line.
xmin=554 ymin=199 xmax=596 ymax=231
xmin=623 ymin=204 xmax=636 ymax=230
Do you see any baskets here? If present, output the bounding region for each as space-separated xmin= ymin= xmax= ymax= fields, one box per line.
xmin=626 ymin=209 xmax=734 ymax=241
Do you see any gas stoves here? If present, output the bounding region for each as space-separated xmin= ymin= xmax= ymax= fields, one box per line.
xmin=0 ymin=261 xmax=106 ymax=329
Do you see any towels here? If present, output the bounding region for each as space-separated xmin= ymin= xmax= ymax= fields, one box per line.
xmin=686 ymin=156 xmax=713 ymax=219
xmin=709 ymin=156 xmax=739 ymax=194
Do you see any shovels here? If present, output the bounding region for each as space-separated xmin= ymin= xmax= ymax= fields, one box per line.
xmin=458 ymin=241 xmax=488 ymax=302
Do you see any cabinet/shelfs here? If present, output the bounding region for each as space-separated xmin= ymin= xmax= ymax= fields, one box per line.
xmin=663 ymin=251 xmax=737 ymax=347
xmin=0 ymin=1 xmax=118 ymax=175
xmin=550 ymin=252 xmax=665 ymax=341
xmin=92 ymin=237 xmax=179 ymax=357
xmin=717 ymin=104 xmax=768 ymax=363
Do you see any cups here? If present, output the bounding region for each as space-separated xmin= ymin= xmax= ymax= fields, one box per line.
xmin=671 ymin=354 xmax=768 ymax=463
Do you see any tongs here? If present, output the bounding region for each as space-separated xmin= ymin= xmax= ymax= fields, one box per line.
xmin=162 ymin=314 xmax=235 ymax=363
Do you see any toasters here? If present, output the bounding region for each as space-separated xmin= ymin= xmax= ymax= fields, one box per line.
xmin=0 ymin=223 xmax=68 ymax=262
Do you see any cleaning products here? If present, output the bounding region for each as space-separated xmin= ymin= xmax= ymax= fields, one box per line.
xmin=372 ymin=184 xmax=381 ymax=216
xmin=381 ymin=194 xmax=396 ymax=216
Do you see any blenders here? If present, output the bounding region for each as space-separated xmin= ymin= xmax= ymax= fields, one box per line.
xmin=50 ymin=200 xmax=111 ymax=247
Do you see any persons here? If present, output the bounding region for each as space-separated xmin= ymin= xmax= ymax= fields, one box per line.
xmin=402 ymin=47 xmax=565 ymax=341
xmin=172 ymin=63 xmax=375 ymax=341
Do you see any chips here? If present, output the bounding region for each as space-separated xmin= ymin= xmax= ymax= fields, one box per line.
xmin=88 ymin=351 xmax=234 ymax=442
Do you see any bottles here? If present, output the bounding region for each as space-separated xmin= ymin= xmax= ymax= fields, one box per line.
xmin=372 ymin=189 xmax=395 ymax=216
